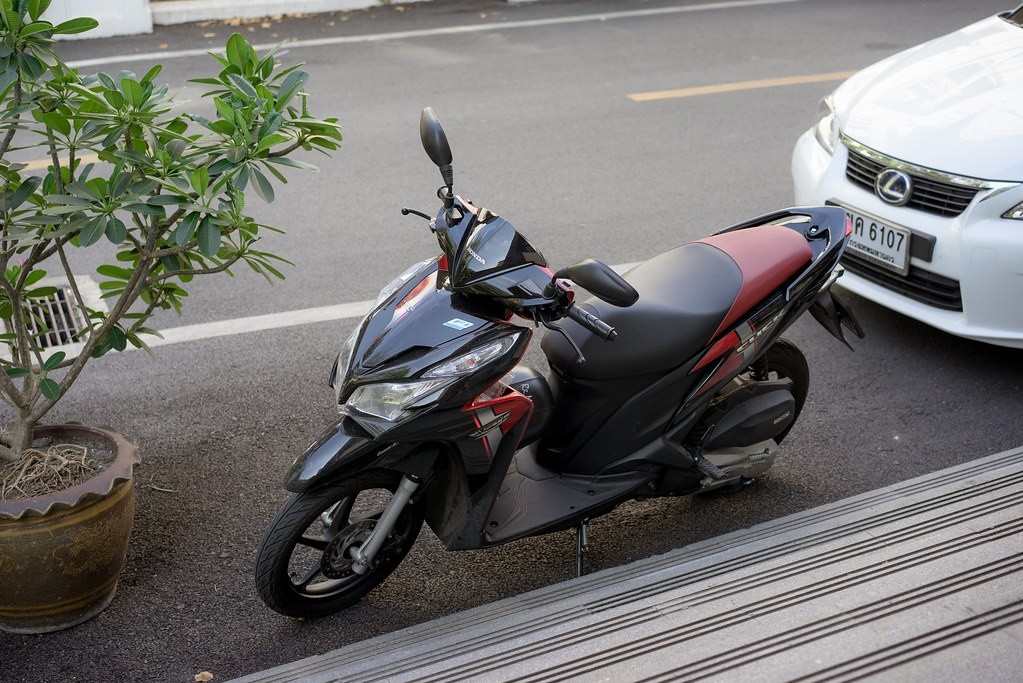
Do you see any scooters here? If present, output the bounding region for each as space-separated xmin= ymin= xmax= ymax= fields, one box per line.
xmin=253 ymin=106 xmax=867 ymax=622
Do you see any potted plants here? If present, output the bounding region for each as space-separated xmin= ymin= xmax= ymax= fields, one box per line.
xmin=0 ymin=0 xmax=344 ymax=635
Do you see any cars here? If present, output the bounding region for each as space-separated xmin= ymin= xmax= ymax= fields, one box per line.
xmin=789 ymin=1 xmax=1023 ymax=355
